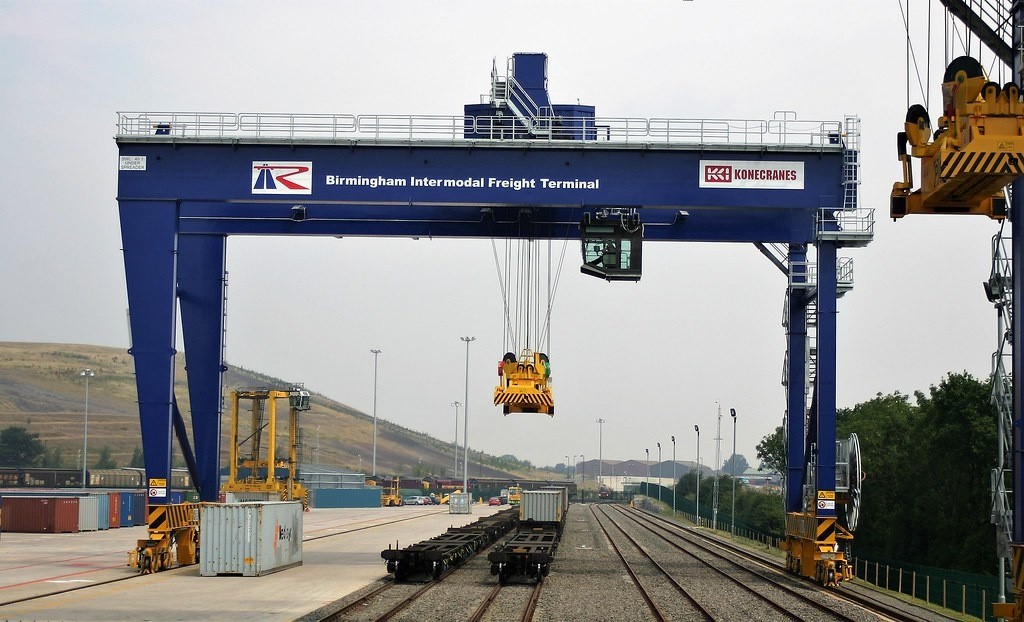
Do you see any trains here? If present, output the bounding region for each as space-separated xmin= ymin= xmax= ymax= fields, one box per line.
xmin=599 ymin=485 xmax=613 ymax=499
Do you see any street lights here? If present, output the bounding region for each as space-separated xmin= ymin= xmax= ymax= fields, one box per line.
xmin=371 ymin=349 xmax=381 ymax=476
xmin=694 ymin=425 xmax=699 ymax=525
xmin=671 ymin=435 xmax=676 ymax=516
xmin=730 ymin=408 xmax=737 ymax=537
xmin=460 ymin=334 xmax=477 ymax=493
xmin=80 ymin=369 xmax=95 ymax=487
xmin=573 ymin=456 xmax=576 ymax=481
xmin=596 ymin=417 xmax=605 ymax=491
xmin=646 ymin=448 xmax=649 ymax=507
xmin=452 ymin=400 xmax=464 ymax=486
xmin=581 ymin=454 xmax=584 ymax=482
xmin=565 ymin=456 xmax=569 ymax=477
xmin=658 ymin=442 xmax=662 ymax=510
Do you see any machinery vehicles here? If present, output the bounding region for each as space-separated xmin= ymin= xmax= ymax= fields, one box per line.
xmin=380 ymin=478 xmax=403 ymax=506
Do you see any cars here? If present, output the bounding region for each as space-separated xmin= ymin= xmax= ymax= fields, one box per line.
xmin=489 ymin=497 xmax=501 ymax=506
xmin=404 ymin=496 xmax=432 ymax=506
